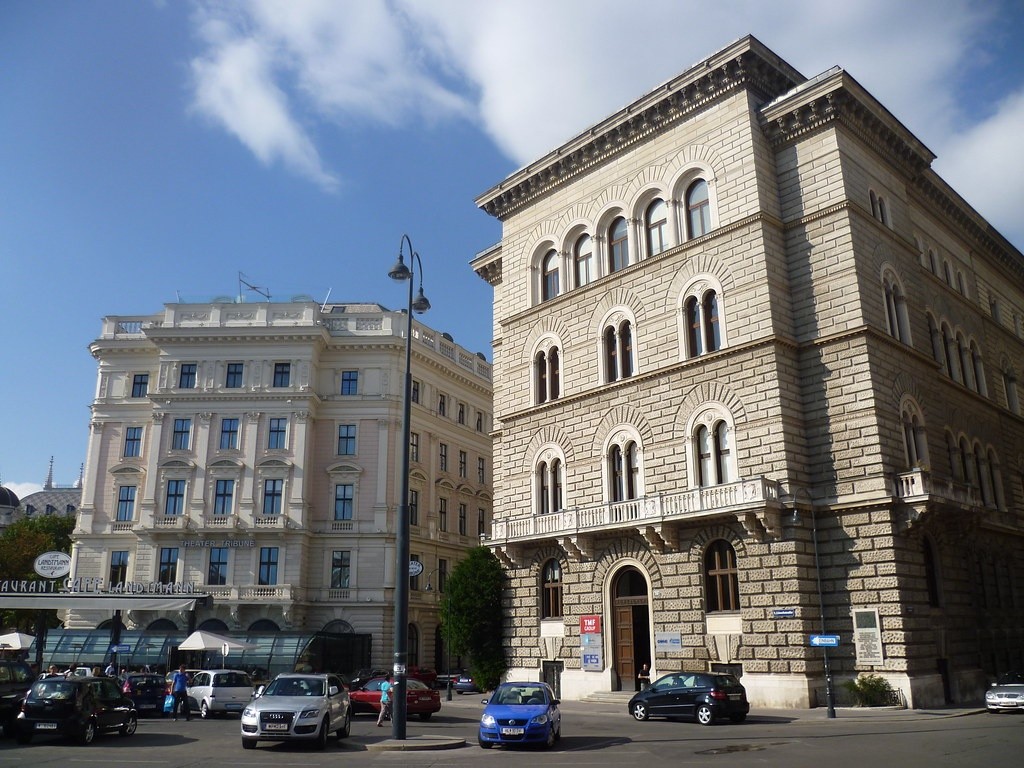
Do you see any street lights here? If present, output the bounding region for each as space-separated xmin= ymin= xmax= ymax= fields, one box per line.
xmin=792 ymin=485 xmax=838 ymax=719
xmin=425 ymin=568 xmax=458 ymax=701
xmin=384 ymin=233 xmax=432 ymax=735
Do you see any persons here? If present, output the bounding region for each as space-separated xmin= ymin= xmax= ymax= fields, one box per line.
xmin=638 ymin=663 xmax=651 ymax=691
xmin=42 ymin=662 xmax=169 ymax=684
xmin=169 ymin=665 xmax=191 ymax=722
xmin=377 ymin=674 xmax=393 ymax=726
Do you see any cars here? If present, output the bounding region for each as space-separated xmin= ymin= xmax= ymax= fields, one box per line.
xmin=626 ymin=671 xmax=751 ymax=725
xmin=186 ymin=669 xmax=254 ymax=720
xmin=124 ymin=673 xmax=170 ymax=716
xmin=453 ymin=673 xmax=482 ymax=694
xmin=0 ymin=660 xmax=36 ymax=747
xmin=985 ymin=672 xmax=1023 ymax=717
xmin=348 ymin=675 xmax=441 ymax=723
xmin=18 ymin=674 xmax=138 ymax=747
xmin=476 ymin=680 xmax=563 ymax=754
xmin=163 ymin=669 xmax=212 ymax=703
xmin=36 ymin=672 xmax=65 ymax=683
xmin=63 ymin=667 xmax=92 ymax=679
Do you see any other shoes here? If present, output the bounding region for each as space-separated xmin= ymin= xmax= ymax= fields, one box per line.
xmin=377 ymin=724 xmax=383 ymax=727
xmin=172 ymin=717 xmax=194 ymax=721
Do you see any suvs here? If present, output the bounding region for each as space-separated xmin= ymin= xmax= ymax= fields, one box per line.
xmin=241 ymin=671 xmax=352 ymax=749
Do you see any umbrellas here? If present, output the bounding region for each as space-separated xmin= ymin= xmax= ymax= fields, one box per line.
xmin=0 ymin=632 xmax=36 ymax=650
xmin=177 ymin=630 xmax=259 ymax=670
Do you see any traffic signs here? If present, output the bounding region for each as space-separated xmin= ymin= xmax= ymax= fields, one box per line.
xmin=809 ymin=632 xmax=840 ymax=648
xmin=109 ymin=644 xmax=130 ymax=654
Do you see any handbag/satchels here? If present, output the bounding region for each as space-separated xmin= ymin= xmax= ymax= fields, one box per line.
xmin=163 ymin=694 xmax=175 ymax=712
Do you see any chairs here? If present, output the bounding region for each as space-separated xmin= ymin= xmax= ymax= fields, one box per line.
xmin=232 ymin=676 xmax=245 ymax=686
xmin=673 ymin=679 xmax=686 ymax=688
xmin=503 ymin=691 xmax=520 ymax=704
xmin=285 ymin=684 xmax=304 ymax=696
xmin=532 ymin=691 xmax=546 ymax=704
xmin=98 ymin=684 xmax=110 ymax=698
xmin=212 ymin=677 xmax=221 ymax=687
xmin=223 ymin=678 xmax=234 ymax=687
